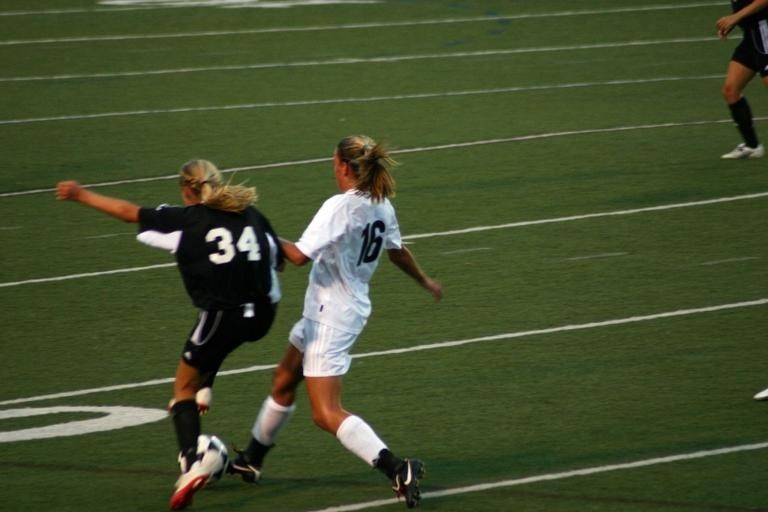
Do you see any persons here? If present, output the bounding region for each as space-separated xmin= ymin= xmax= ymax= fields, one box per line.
xmin=54 ymin=156 xmax=286 ymax=510
xmin=712 ymin=1 xmax=768 ymax=164
xmin=221 ymin=135 xmax=443 ymax=509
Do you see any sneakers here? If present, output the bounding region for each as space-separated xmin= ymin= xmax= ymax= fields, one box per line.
xmin=391 ymin=457 xmax=425 ymax=510
xmin=217 ymin=453 xmax=263 ymax=484
xmin=167 ymin=387 xmax=214 ymax=416
xmin=167 ymin=449 xmax=225 ymax=511
xmin=719 ymin=141 xmax=765 ymax=160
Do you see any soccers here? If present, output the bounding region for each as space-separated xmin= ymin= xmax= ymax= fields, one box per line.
xmin=178 ymin=433 xmax=229 ymax=486
